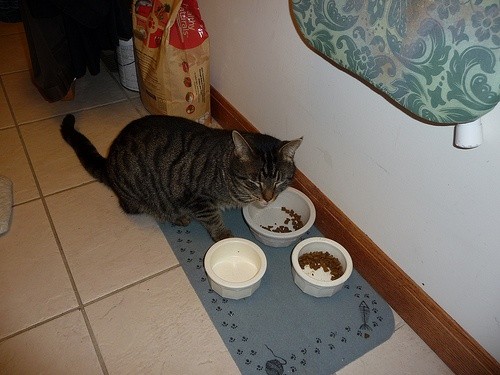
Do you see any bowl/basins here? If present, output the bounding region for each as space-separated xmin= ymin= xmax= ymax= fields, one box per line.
xmin=241 ymin=186 xmax=317 ymax=248
xmin=290 ymin=237 xmax=353 ymax=297
xmin=203 ymin=237 xmax=267 ymax=300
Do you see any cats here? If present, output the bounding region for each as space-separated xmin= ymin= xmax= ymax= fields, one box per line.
xmin=59 ymin=114 xmax=303 ymax=242
